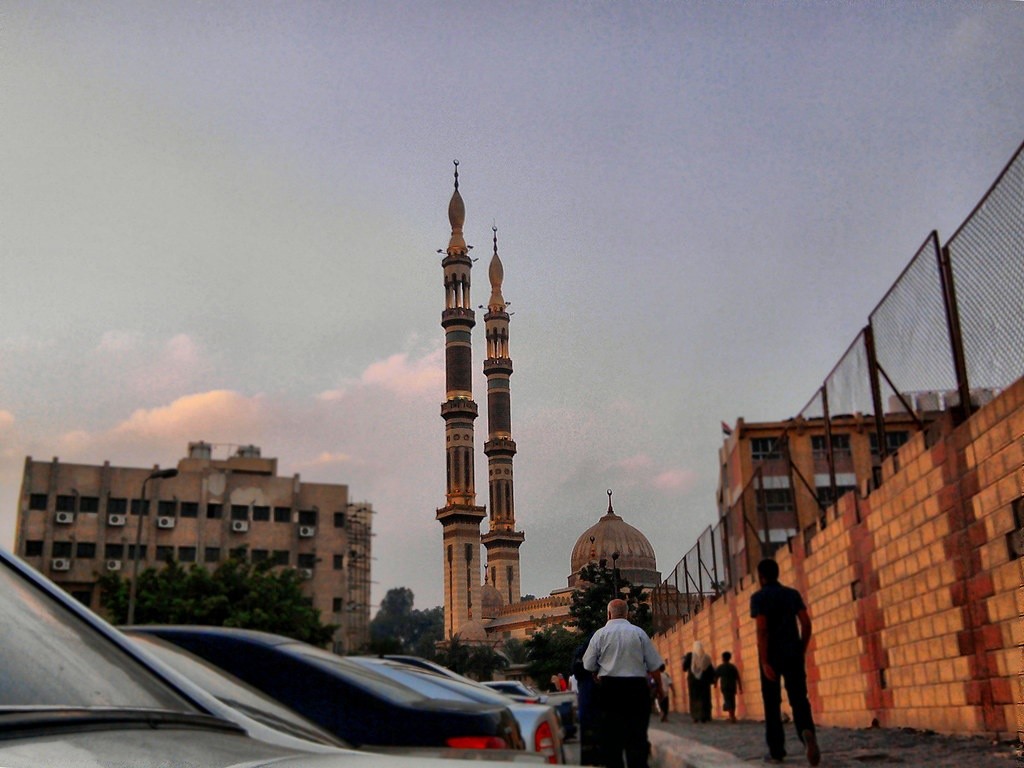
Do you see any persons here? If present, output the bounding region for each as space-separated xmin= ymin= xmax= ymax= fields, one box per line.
xmin=750 ymin=558 xmax=821 ymax=767
xmin=714 ymin=651 xmax=744 ymax=724
xmin=582 ymin=599 xmax=664 ymax=768
xmin=654 ymin=664 xmax=676 ymax=722
xmin=549 ymin=672 xmax=580 ymax=695
xmin=682 ymin=641 xmax=715 ymax=724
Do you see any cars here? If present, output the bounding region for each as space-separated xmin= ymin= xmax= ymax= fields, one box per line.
xmin=346 ymin=649 xmax=576 ymax=766
xmin=0 ymin=550 xmax=559 ymax=768
xmin=112 ymin=625 xmax=524 ymax=755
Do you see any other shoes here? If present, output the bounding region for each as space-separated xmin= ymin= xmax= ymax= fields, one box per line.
xmin=802 ymin=729 xmax=821 ymax=766
xmin=727 ymin=717 xmax=736 ymax=724
xmin=661 ymin=713 xmax=667 ymax=721
xmin=764 ymin=753 xmax=783 ymax=764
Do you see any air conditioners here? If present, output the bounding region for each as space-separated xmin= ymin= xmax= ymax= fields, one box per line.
xmin=53 ymin=558 xmax=70 ymax=569
xmin=233 ymin=520 xmax=249 ymax=532
xmin=301 ymin=569 xmax=312 ymax=578
xmin=109 ymin=514 xmax=125 ymax=525
xmin=107 ymin=560 xmax=121 ymax=570
xmin=56 ymin=513 xmax=73 ymax=523
xmin=158 ymin=516 xmax=175 ymax=528
xmin=300 ymin=526 xmax=315 ymax=536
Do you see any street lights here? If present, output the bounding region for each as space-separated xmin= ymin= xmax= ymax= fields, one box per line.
xmin=610 ymin=550 xmax=621 ymax=598
xmin=127 ymin=466 xmax=181 ymax=623
